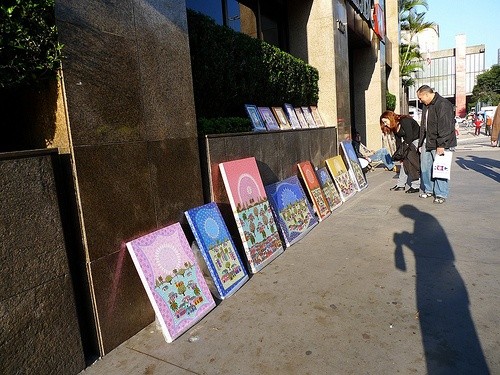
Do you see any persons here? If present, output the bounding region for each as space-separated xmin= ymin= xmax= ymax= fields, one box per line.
xmin=379 ymin=110 xmax=423 ymax=194
xmin=491 ymin=100 xmax=500 ymax=149
xmin=455 ymin=117 xmax=460 ymax=140
xmin=416 ymin=84 xmax=456 ymax=204
xmin=473 ymin=117 xmax=482 ymax=136
xmin=483 ymin=115 xmax=492 ymax=136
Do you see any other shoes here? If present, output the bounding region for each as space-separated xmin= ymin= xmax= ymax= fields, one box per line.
xmin=385 ymin=167 xmax=389 ymax=171
xmin=393 ymin=166 xmax=400 ymax=172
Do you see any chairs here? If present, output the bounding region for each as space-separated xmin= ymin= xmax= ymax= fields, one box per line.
xmin=352 ymin=140 xmax=384 ymax=172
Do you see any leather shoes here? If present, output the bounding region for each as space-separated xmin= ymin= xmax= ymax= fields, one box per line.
xmin=390 ymin=185 xmax=405 ymax=191
xmin=405 ymin=187 xmax=419 ymax=193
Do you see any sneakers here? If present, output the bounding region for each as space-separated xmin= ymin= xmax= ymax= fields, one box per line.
xmin=419 ymin=192 xmax=432 ymax=199
xmin=433 ymin=196 xmax=446 ymax=204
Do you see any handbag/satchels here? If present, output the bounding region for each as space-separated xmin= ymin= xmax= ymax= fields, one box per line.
xmin=431 ymin=150 xmax=452 ymax=180
xmin=391 ymin=144 xmax=410 ymax=161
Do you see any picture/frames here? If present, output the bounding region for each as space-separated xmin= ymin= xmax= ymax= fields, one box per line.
xmin=257 ymin=107 xmax=280 ymax=131
xmin=285 ymin=104 xmax=301 ymax=129
xmin=310 ymin=106 xmax=325 ymax=128
xmin=301 ymin=107 xmax=317 ymax=128
xmin=294 ymin=108 xmax=309 ymax=129
xmin=245 ymin=104 xmax=267 ymax=131
xmin=272 ymin=107 xmax=292 ymax=130
